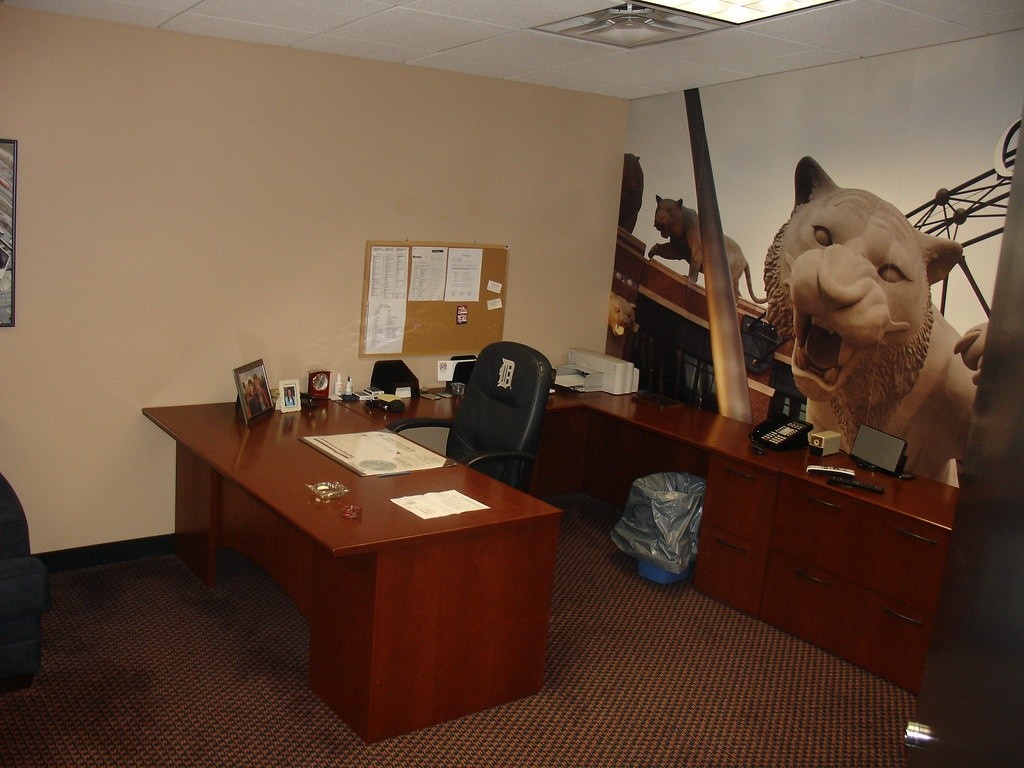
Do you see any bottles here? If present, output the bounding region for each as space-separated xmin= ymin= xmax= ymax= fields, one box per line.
xmin=335 ymin=373 xmax=342 ymax=396
xmin=345 ymin=376 xmax=352 ymax=395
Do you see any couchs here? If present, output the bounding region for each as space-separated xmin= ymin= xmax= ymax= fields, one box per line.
xmin=0 ymin=473 xmax=49 ymax=693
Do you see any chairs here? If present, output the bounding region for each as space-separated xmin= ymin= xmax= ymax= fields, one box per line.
xmin=392 ymin=341 xmax=552 ymax=491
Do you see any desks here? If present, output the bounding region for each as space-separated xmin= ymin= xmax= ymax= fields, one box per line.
xmin=142 ymin=397 xmax=564 ymax=745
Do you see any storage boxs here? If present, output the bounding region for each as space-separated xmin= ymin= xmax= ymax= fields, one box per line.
xmin=353 ymin=387 xmax=384 ymax=401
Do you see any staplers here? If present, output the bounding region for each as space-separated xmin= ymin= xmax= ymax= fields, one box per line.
xmin=301 ymin=393 xmax=316 ymax=409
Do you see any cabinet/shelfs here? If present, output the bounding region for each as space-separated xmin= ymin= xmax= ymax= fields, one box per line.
xmin=333 ymin=383 xmax=962 ymax=698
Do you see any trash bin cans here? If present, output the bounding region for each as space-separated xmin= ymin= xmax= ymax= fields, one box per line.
xmin=629 ymin=468 xmax=707 ymax=586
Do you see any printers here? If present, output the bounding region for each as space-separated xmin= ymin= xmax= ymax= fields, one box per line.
xmin=555 ymin=348 xmax=639 ymax=395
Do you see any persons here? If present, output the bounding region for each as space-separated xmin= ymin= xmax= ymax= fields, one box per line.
xmin=245 ymin=374 xmax=270 ymax=415
xmin=285 ymin=388 xmax=296 ymax=405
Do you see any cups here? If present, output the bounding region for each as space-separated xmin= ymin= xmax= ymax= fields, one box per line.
xmin=451 ymin=382 xmax=465 ymax=396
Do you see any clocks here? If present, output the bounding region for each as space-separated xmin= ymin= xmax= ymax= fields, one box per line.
xmin=308 ymin=369 xmax=331 ymax=401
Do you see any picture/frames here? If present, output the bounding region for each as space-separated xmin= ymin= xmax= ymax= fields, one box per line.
xmin=231 ymin=359 xmax=275 ymax=426
xmin=0 ymin=138 xmax=17 ymax=328
xmin=278 ymin=379 xmax=301 ymax=413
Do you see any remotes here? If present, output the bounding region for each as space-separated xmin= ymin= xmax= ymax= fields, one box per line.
xmin=827 ymin=476 xmax=884 ymax=494
xmin=805 ymin=465 xmax=855 ymax=478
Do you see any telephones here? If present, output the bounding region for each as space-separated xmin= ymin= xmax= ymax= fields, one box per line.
xmin=748 ymin=411 xmax=814 ymax=453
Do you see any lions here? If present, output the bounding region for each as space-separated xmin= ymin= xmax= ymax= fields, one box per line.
xmin=647 ymin=194 xmax=769 ymax=308
xmin=605 ymin=292 xmax=637 ymax=359
xmin=763 ymin=154 xmax=987 ymax=490
